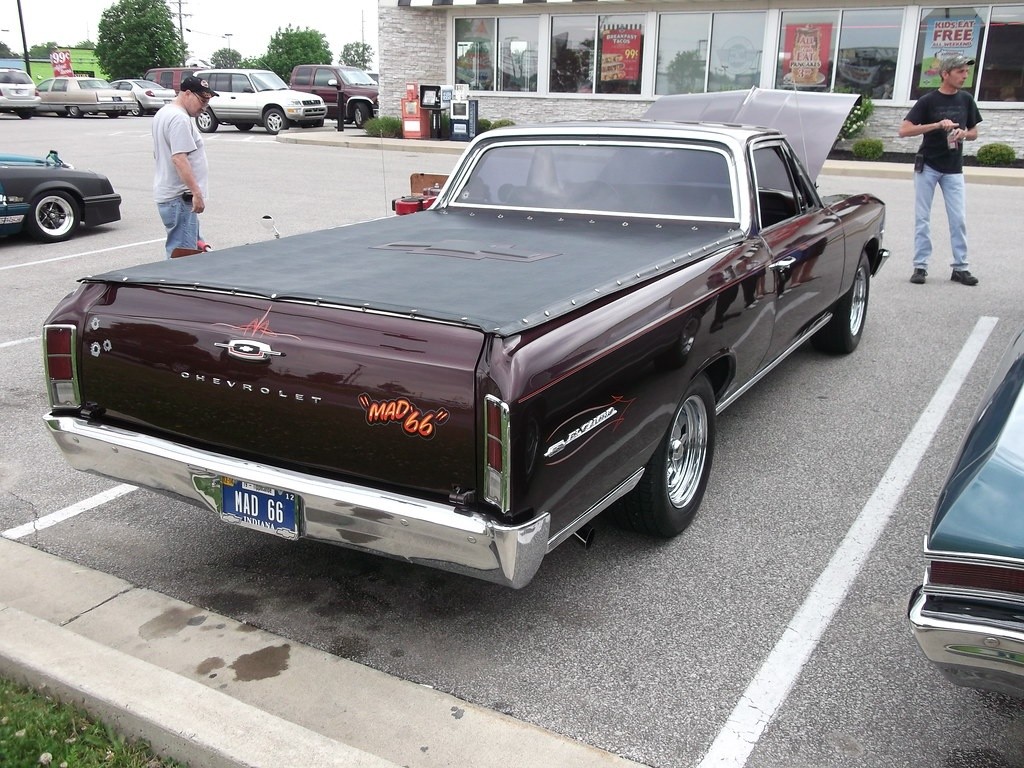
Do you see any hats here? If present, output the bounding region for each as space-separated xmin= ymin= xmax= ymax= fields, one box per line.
xmin=939 ymin=55 xmax=974 ymax=73
xmin=180 ymin=76 xmax=220 ymax=99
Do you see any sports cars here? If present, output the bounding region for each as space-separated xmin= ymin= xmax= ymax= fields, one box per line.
xmin=41 ymin=86 xmax=894 ymax=589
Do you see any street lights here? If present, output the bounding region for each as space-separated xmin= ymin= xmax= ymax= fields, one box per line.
xmin=225 ymin=34 xmax=233 ymax=68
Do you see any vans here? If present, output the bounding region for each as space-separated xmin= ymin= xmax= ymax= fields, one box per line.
xmin=141 ymin=67 xmax=209 ymax=95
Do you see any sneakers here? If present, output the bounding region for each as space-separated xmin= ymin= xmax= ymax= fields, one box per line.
xmin=950 ymin=269 xmax=978 ymax=285
xmin=910 ymin=268 xmax=928 ymax=284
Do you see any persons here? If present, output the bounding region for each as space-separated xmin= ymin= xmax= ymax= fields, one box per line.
xmin=150 ymin=76 xmax=219 ymax=260
xmin=898 ymin=54 xmax=983 ymax=285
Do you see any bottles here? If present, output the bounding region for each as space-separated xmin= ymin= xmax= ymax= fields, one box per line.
xmin=947 ymin=129 xmax=959 ymax=152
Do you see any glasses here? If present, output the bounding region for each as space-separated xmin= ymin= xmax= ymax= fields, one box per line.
xmin=192 ymin=91 xmax=209 ymax=104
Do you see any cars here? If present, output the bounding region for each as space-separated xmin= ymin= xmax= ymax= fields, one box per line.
xmin=32 ymin=76 xmax=138 ymax=118
xmin=907 ymin=331 xmax=1024 ymax=698
xmin=109 ymin=79 xmax=177 ymax=117
xmin=0 ymin=153 xmax=122 ymax=244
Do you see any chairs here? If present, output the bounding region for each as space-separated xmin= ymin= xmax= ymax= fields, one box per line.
xmin=568 ymin=179 xmax=623 ymax=213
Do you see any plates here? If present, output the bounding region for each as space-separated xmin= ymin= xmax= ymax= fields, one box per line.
xmin=783 ymin=72 xmax=826 ymax=86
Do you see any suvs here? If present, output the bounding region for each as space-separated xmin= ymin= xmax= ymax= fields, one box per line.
xmin=0 ymin=66 xmax=41 ymax=119
xmin=187 ymin=69 xmax=328 ymax=134
xmin=288 ymin=64 xmax=378 ymax=129
